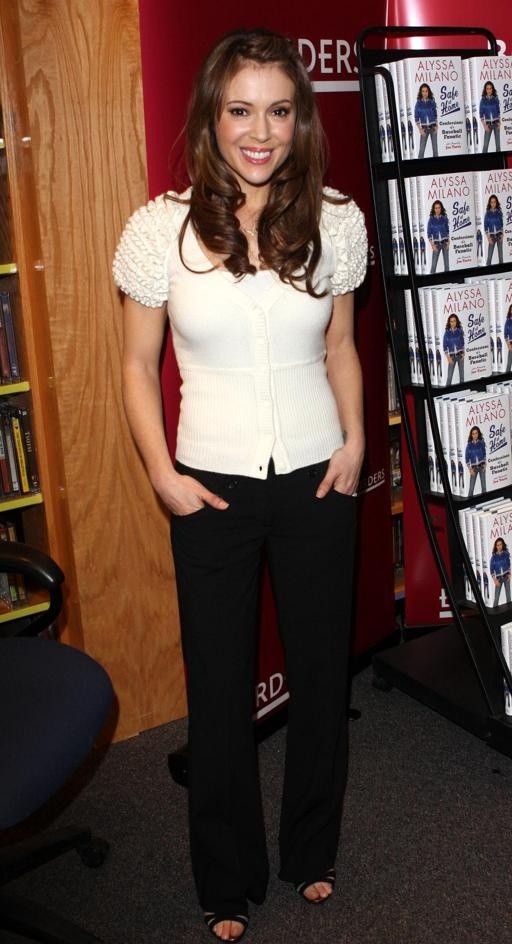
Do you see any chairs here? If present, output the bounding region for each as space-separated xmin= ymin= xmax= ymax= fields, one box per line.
xmin=0 ymin=534 xmax=125 ymax=871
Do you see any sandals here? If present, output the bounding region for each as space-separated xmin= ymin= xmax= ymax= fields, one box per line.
xmin=203 ymin=910 xmax=249 ymax=943
xmin=295 ymin=867 xmax=336 ymax=906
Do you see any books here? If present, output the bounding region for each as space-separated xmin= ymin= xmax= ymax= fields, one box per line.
xmin=458 ymin=496 xmax=509 ymax=609
xmin=422 ymin=380 xmax=510 ymax=499
xmin=1 ymin=286 xmax=43 ymax=616
xmin=470 ymin=169 xmax=512 ymax=266
xmin=374 ymin=57 xmax=512 ymax=163
xmin=415 ymin=169 xmax=476 ymax=276
xmin=417 ymin=271 xmax=509 ymax=387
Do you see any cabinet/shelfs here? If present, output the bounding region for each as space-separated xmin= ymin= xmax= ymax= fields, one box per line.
xmin=0 ymin=39 xmax=64 ymax=625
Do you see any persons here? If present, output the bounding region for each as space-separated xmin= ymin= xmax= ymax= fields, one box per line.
xmin=484 ymin=194 xmax=505 ymax=266
xmin=415 ymin=83 xmax=441 ymax=160
xmin=479 ymin=80 xmax=501 ymax=154
xmin=110 ymin=26 xmax=370 ymax=943
xmin=427 ymin=198 xmax=450 ymax=274
